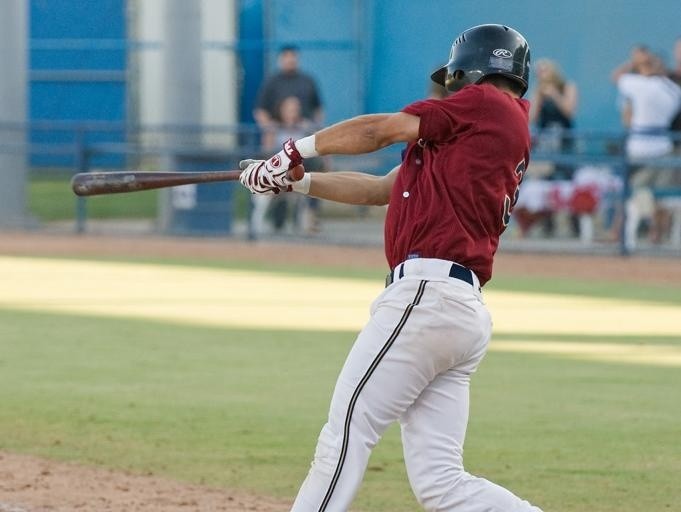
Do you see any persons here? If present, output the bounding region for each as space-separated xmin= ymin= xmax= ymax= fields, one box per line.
xmin=251 ymin=43 xmax=325 ymax=215
xmin=529 ymin=58 xmax=584 ymax=237
xmin=240 ymin=21 xmax=550 ymax=511
xmin=608 ymin=38 xmax=680 ymax=258
xmin=264 ymin=95 xmax=325 ymax=236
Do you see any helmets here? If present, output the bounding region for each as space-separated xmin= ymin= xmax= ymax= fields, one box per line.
xmin=432 ymin=25 xmax=531 ymax=98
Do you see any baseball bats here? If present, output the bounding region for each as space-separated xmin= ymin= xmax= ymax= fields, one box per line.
xmin=73 ymin=163 xmax=304 ymax=196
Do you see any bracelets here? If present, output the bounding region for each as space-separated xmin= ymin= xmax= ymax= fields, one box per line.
xmin=295 ymin=170 xmax=312 ymax=195
xmin=297 ymin=131 xmax=320 ymax=160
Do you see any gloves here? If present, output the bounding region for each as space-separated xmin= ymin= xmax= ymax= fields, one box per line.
xmin=239 ymin=138 xmax=304 ymax=196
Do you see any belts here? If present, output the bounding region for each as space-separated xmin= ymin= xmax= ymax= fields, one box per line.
xmin=385 ymin=263 xmax=474 ymax=284
